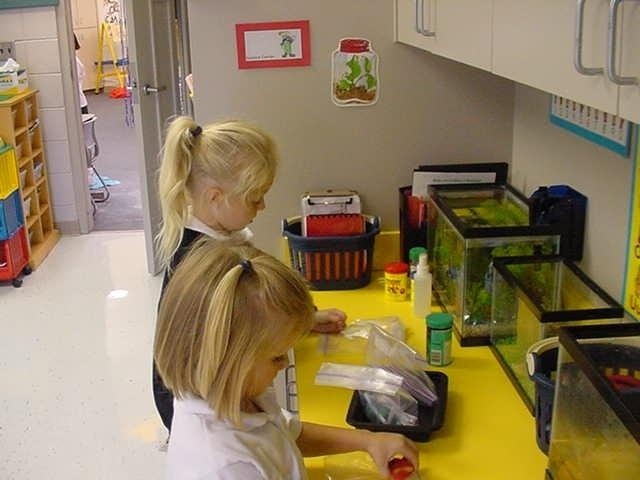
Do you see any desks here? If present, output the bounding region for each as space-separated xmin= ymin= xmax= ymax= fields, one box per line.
xmin=278 ymin=231 xmax=549 ymax=479
xmin=80 ymin=113 xmax=105 ymax=199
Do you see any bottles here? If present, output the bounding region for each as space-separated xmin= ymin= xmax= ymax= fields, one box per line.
xmin=408 ymin=246 xmax=427 ymax=302
xmin=412 ymin=255 xmax=434 ymax=318
xmin=331 ymin=37 xmax=382 ymax=108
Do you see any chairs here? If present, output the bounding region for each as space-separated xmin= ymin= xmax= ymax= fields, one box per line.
xmin=81 ymin=115 xmax=110 ymax=216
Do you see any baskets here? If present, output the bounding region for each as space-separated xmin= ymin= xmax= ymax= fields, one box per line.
xmin=280 ymin=212 xmax=383 ymax=292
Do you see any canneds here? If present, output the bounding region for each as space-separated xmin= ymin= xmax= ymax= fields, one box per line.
xmin=384 ymin=261 xmax=409 ymax=301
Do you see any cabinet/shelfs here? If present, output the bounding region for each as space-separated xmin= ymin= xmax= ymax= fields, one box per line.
xmin=491 ymin=0 xmax=639 ymax=126
xmin=1 ymin=89 xmax=62 ymax=272
xmin=393 ymin=0 xmax=492 ymax=74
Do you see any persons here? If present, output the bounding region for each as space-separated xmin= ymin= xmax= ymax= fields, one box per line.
xmin=154 ymin=232 xmax=421 ymax=480
xmin=71 ymin=30 xmax=88 ymax=118
xmin=147 ymin=113 xmax=348 ymax=440
